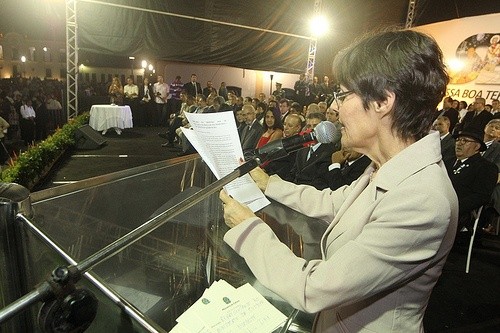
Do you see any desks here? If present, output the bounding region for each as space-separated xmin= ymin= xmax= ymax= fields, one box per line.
xmin=88 ymin=105 xmax=133 ymax=135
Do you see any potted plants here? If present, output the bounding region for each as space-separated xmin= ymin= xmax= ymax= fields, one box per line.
xmin=0 ymin=112 xmax=91 ymax=190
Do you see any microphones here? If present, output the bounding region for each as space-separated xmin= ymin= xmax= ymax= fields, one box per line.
xmin=244 ymin=121 xmax=338 ymax=162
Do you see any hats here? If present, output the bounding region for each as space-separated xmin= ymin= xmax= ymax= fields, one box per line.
xmin=451 ymin=127 xmax=487 ymax=152
xmin=275 ymin=82 xmax=283 ymax=86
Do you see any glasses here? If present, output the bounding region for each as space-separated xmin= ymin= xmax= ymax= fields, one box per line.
xmin=332 ymin=89 xmax=356 ymax=108
xmin=473 ymin=101 xmax=480 ymax=104
xmin=456 ymin=137 xmax=478 ymax=145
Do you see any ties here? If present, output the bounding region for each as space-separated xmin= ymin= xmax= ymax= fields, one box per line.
xmin=452 ymin=160 xmax=463 ymax=170
xmin=341 ymin=162 xmax=349 ymax=176
xmin=310 ymin=147 xmax=315 ymax=158
xmin=473 ymin=111 xmax=478 ymax=119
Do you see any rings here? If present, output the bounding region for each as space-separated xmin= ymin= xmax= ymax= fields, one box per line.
xmin=221 ymin=202 xmax=225 ymax=211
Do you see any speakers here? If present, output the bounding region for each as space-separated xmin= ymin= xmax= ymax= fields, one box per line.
xmin=70 ymin=123 xmax=108 ymax=150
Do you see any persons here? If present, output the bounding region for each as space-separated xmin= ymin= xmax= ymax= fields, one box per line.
xmin=108 ymin=77 xmax=123 ymax=106
xmin=434 ymin=97 xmax=500 ymax=275
xmin=153 ymin=76 xmax=170 ymax=126
xmin=0 ymin=76 xmax=111 ymax=166
xmin=123 ymin=77 xmax=139 ymax=127
xmin=219 ymin=29 xmax=459 ymax=333
xmin=160 ymin=73 xmax=373 ymax=213
xmin=138 ymin=77 xmax=155 ymax=127
